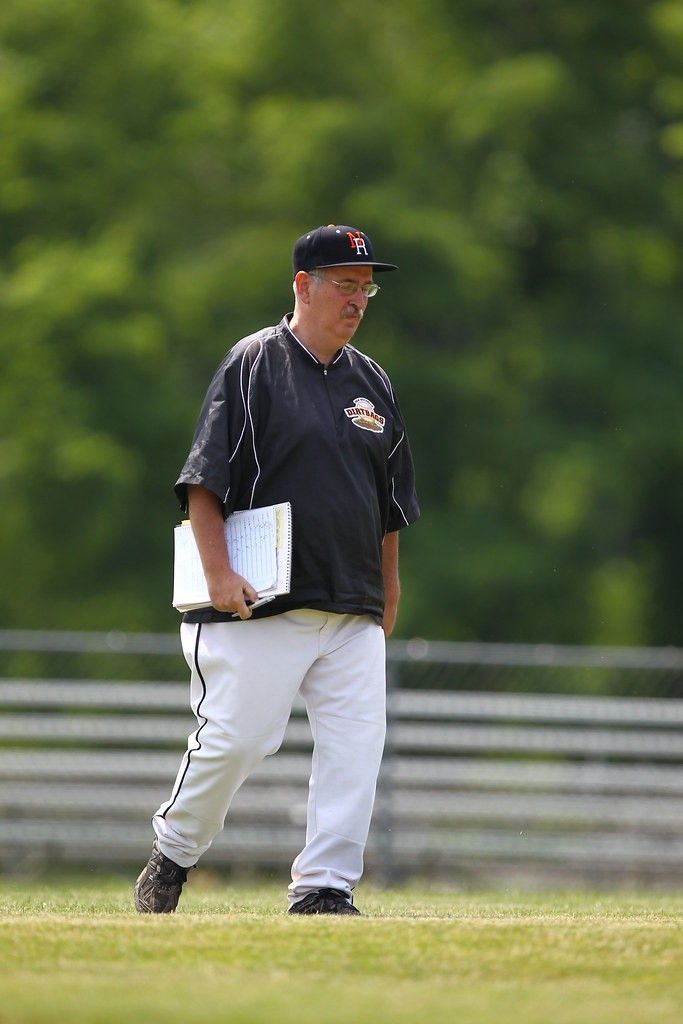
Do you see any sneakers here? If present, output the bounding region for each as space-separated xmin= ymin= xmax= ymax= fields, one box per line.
xmin=133 ymin=834 xmax=190 ymax=916
xmin=286 ymin=887 xmax=365 ymax=916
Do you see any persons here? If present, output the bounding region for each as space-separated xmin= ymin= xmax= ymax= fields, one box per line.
xmin=134 ymin=223 xmax=420 ymax=915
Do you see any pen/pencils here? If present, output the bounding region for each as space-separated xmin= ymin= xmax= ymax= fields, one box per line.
xmin=232 ymin=595 xmax=275 ymax=617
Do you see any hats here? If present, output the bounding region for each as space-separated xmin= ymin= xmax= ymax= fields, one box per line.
xmin=291 ymin=223 xmax=400 ymax=277
